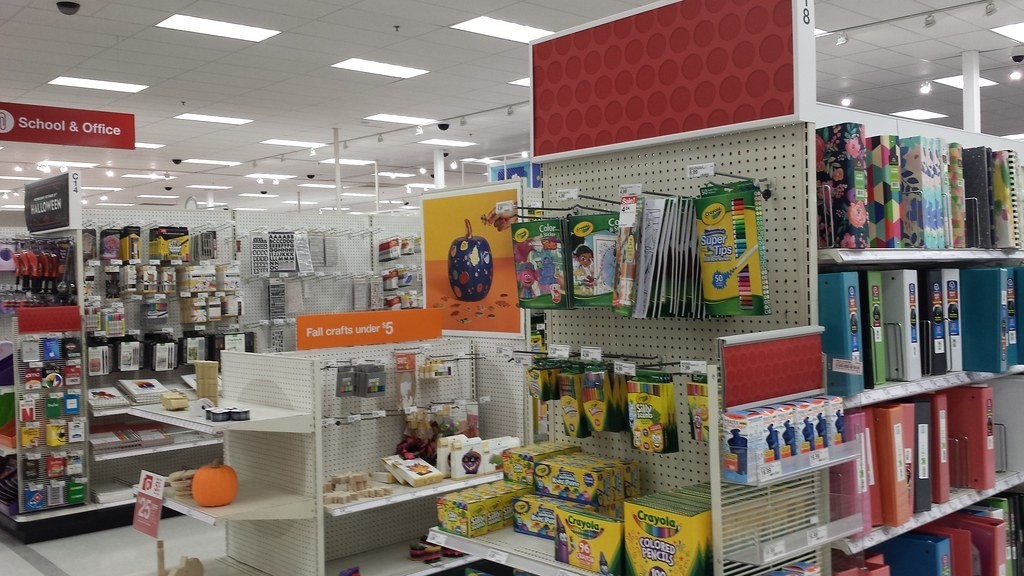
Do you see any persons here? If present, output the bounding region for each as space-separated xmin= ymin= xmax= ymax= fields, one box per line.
xmin=487 ymin=202 xmax=518 ymax=232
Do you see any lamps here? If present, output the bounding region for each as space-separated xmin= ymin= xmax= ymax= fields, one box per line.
xmin=986 ymin=0 xmax=998 ymax=16
xmin=253 ymin=160 xmax=258 ymax=167
xmin=310 ymin=148 xmax=316 ymax=157
xmin=925 ymin=14 xmax=936 ymax=27
xmin=343 ymin=142 xmax=348 ymax=149
xmin=378 ymin=134 xmax=383 ymax=142
xmin=416 ymin=126 xmax=423 ymax=135
xmin=281 ymin=154 xmax=286 ymax=162
xmin=836 ymin=31 xmax=847 ymax=45
xmin=505 ymin=106 xmax=513 ymax=116
xmin=460 ymin=116 xmax=467 ymax=126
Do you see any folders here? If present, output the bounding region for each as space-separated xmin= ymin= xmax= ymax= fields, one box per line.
xmin=815 ymin=121 xmax=1024 ymax=576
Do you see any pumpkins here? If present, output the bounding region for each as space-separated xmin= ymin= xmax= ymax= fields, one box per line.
xmin=192 ymin=457 xmax=237 ymax=507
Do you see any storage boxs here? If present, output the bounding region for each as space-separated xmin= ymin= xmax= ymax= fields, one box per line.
xmin=721 ymin=394 xmax=846 ymax=485
xmin=380 ymin=434 xmax=804 ymax=576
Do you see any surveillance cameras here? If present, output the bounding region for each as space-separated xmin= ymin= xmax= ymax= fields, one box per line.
xmin=431 ymin=174 xmax=433 ymax=178
xmin=165 ymin=187 xmax=172 ymax=191
xmin=173 ymin=159 xmax=182 ymax=164
xmin=224 ymin=208 xmax=228 ymax=210
xmin=261 ymin=191 xmax=267 ymax=194
xmin=307 ymin=175 xmax=315 ymax=179
xmin=1012 ymin=55 xmax=1024 ymax=62
xmin=443 ymin=153 xmax=448 ymax=157
xmin=57 ymin=2 xmax=80 ymax=16
xmin=438 ymin=124 xmax=449 ymax=130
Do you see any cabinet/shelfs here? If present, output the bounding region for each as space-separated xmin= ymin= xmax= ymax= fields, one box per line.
xmin=129 ymin=338 xmax=482 ymax=576
xmin=818 ymin=248 xmax=1023 ymax=556
xmin=0 ymin=385 xmax=224 ymax=546
xmin=720 ymin=438 xmax=864 ymax=566
